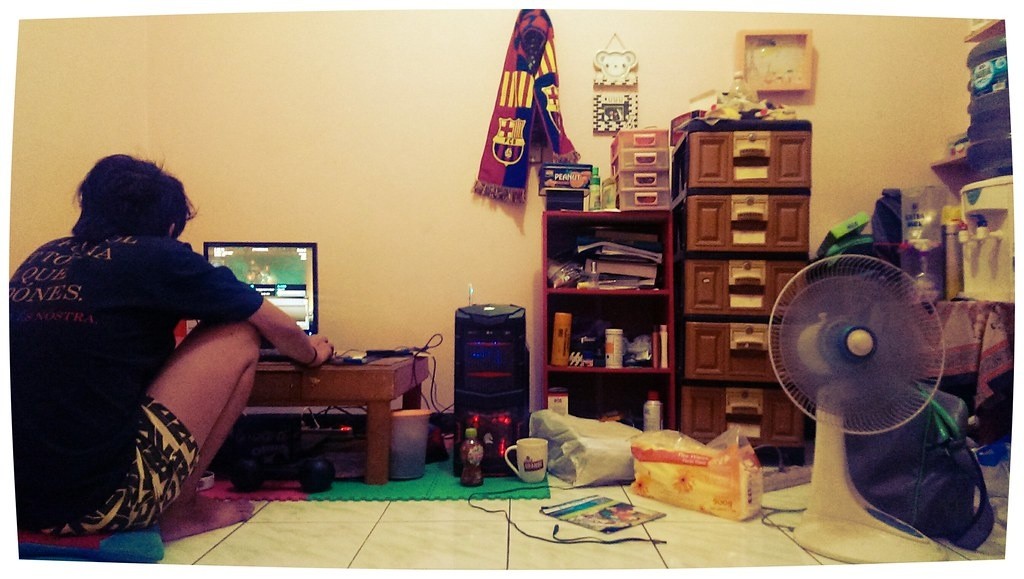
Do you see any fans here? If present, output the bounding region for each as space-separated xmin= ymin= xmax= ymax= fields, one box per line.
xmin=768 ymin=253 xmax=946 ymax=563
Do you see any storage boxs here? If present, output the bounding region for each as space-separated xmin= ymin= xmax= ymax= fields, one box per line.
xmin=537 ymin=129 xmax=670 ymax=209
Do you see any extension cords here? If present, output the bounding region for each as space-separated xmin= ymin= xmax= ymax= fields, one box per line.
xmin=301 ymin=426 xmax=353 ymax=441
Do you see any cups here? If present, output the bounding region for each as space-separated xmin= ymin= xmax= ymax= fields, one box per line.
xmin=504 ymin=438 xmax=551 ymax=484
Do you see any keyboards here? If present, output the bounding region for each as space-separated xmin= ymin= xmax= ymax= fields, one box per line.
xmin=258 ymin=348 xmax=289 ymax=362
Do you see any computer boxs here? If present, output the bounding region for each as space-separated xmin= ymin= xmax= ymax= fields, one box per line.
xmin=455 ymin=303 xmax=531 ymax=475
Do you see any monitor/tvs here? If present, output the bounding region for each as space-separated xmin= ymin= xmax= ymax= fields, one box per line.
xmin=204 ymin=242 xmax=318 ymax=350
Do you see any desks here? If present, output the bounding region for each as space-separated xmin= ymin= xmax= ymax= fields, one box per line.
xmin=253 ymin=352 xmax=430 ymax=484
xmin=929 ymin=298 xmax=1014 ymax=406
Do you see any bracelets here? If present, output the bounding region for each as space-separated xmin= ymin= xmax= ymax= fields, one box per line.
xmin=307 ymin=346 xmax=317 ymax=365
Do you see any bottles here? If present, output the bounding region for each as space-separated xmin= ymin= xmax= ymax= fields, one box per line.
xmin=965 ymin=31 xmax=1013 ymax=182
xmin=460 ymin=428 xmax=484 ymax=486
xmin=602 ymin=329 xmax=626 ymax=369
xmin=643 ymin=391 xmax=663 ymax=432
xmin=729 ymin=72 xmax=752 ymax=102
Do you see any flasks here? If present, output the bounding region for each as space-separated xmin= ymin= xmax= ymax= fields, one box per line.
xmin=943 ymin=221 xmax=963 ymax=299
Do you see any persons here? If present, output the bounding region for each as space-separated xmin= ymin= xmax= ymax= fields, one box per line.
xmin=9 ymin=154 xmax=335 ymax=542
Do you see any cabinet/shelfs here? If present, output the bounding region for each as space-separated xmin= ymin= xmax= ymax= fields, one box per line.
xmin=541 ymin=209 xmax=675 ymax=431
xmin=683 ymin=128 xmax=812 ymax=468
